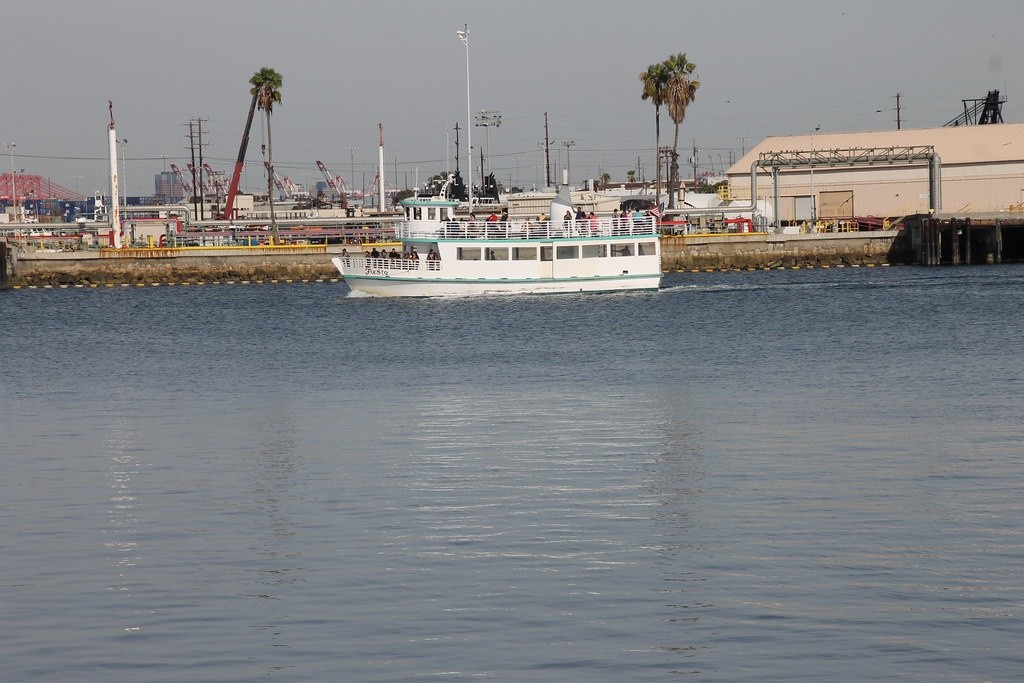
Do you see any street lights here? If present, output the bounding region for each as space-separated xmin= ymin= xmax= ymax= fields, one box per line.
xmin=456 ymin=23 xmax=473 ymax=216
xmin=116 ymin=138 xmax=127 ymax=219
xmin=562 ymin=140 xmax=575 ymax=184
xmin=4 ymin=142 xmax=17 ymax=236
xmin=475 ymin=108 xmax=503 ymax=192
xmin=346 ymin=146 xmax=358 ymax=193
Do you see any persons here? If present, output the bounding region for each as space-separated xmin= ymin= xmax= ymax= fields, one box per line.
xmin=441 ymin=213 xmax=478 ymax=238
xmin=92 ymin=234 xmax=99 ymax=248
xmin=612 ymin=245 xmax=631 ymax=256
xmin=610 ymin=207 xmax=652 ymax=235
xmin=426 ymin=248 xmax=438 ymax=270
xmin=482 ymin=212 xmax=499 ymax=240
xmin=521 ymin=212 xmax=548 ymax=239
xmin=563 ymin=207 xmax=601 ymax=238
xmin=639 ymin=244 xmax=646 ymax=255
xmin=341 ymin=248 xmax=351 ymax=267
xmin=707 ymin=218 xmax=718 ymax=234
xmin=363 ymin=247 xmax=419 ymax=269
xmin=500 ymin=211 xmax=508 ymax=238
xmin=490 ymin=250 xmax=496 ymax=260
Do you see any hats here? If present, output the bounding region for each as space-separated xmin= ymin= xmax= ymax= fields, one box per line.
xmin=628 ymin=209 xmax=631 ymax=210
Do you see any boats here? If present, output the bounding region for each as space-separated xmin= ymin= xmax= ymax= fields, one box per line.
xmin=331 ymin=192 xmax=663 ymax=298
